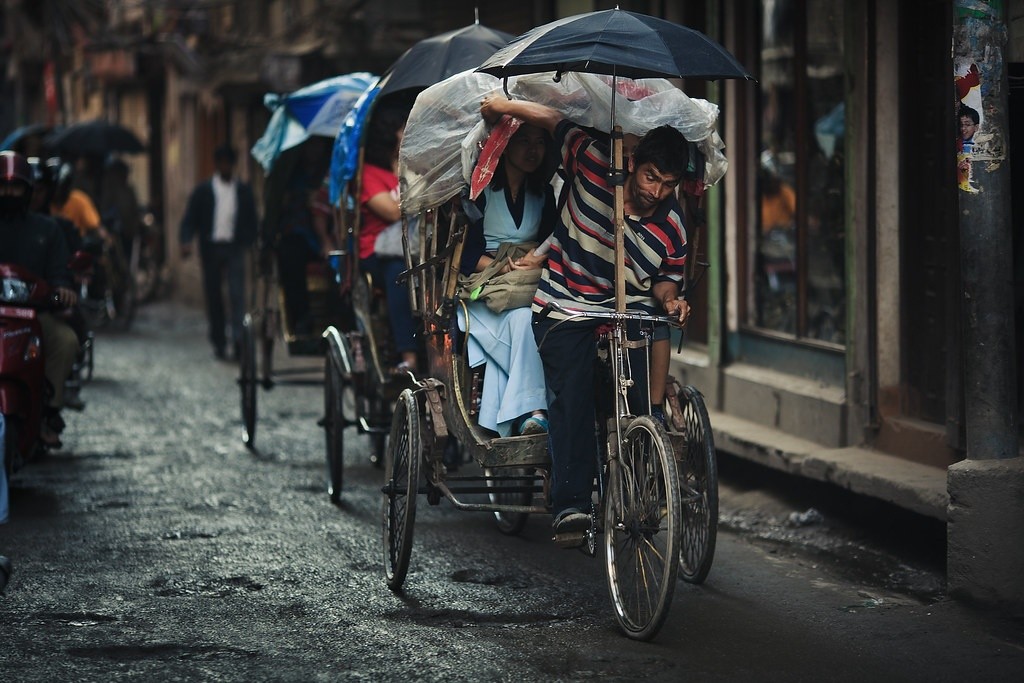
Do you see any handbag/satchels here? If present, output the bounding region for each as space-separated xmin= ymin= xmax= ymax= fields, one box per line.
xmin=455 ymin=241 xmax=544 ymax=314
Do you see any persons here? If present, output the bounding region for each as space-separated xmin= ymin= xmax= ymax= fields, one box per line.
xmin=349 ymin=101 xmax=418 ymax=377
xmin=263 ymin=138 xmax=334 ymax=333
xmin=427 ymin=123 xmax=549 ymax=437
xmin=178 ymin=144 xmax=258 ymax=357
xmin=957 ymin=108 xmax=980 ymax=151
xmin=0 ymin=151 xmax=139 ymax=449
xmin=480 ymin=96 xmax=690 ymax=550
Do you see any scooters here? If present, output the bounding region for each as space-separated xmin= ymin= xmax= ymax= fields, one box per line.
xmin=0 ymin=264 xmax=72 ymax=480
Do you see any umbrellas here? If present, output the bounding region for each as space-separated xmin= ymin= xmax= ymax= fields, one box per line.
xmin=376 ymin=7 xmax=517 ymax=99
xmin=475 ymin=4 xmax=758 ymax=185
xmin=49 ymin=117 xmax=146 ymax=156
xmin=0 ymin=123 xmax=64 ymax=156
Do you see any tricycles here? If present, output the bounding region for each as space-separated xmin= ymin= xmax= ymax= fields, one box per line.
xmin=237 ymin=72 xmax=539 ymax=538
xmin=316 ymin=65 xmax=723 ymax=640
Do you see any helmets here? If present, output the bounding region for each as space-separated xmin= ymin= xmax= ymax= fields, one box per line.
xmin=0 ymin=150 xmax=33 ymax=188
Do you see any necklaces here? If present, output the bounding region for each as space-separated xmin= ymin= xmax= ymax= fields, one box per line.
xmin=512 ymin=188 xmax=518 ymax=199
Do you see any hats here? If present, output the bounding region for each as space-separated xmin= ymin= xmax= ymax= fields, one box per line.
xmin=42 ymin=150 xmax=75 ymax=176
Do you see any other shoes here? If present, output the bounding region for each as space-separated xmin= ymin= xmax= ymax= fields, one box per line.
xmin=388 ymin=362 xmax=420 ymax=380
xmin=551 ymin=513 xmax=590 ymax=549
xmin=518 ymin=417 xmax=549 ymax=436
xmin=41 ymin=423 xmax=60 ymax=447
xmin=213 ymin=347 xmax=226 ymax=358
